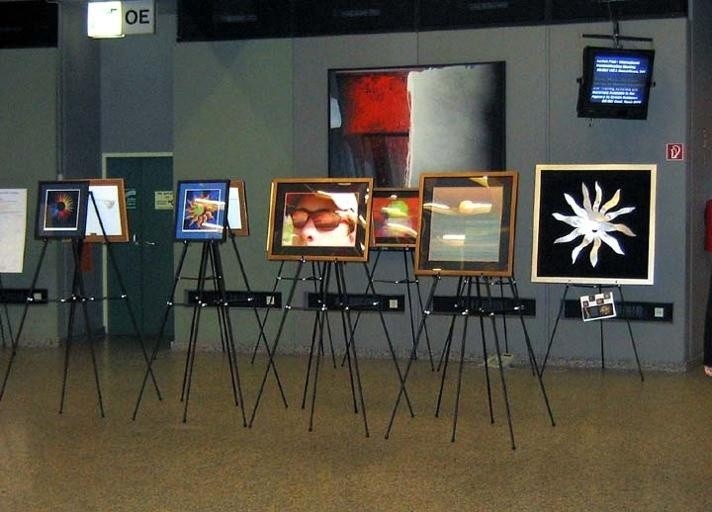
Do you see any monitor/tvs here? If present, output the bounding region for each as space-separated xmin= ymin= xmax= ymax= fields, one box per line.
xmin=576 ymin=45 xmax=655 ymax=121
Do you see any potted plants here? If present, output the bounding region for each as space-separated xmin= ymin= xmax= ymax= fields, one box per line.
xmin=227 ymin=180 xmax=249 ymax=236
xmin=266 ymin=177 xmax=374 ymax=262
xmin=530 ymin=163 xmax=657 ymax=286
xmin=172 ymin=179 xmax=231 ymax=241
xmin=369 ymin=187 xmax=418 ymax=252
xmin=413 ymin=169 xmax=518 ymax=277
xmin=83 ymin=178 xmax=129 ymax=244
xmin=34 ymin=180 xmax=90 ymax=239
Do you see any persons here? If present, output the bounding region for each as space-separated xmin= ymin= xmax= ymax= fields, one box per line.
xmin=285 ymin=192 xmax=357 ymax=247
xmin=703 ymin=198 xmax=712 ymax=378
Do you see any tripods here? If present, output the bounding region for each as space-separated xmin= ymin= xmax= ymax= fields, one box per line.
xmin=1 ymin=192 xmax=554 ymax=452
xmin=539 ymin=285 xmax=644 ymax=381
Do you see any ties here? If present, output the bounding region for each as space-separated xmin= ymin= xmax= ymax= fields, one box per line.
xmin=576 ymin=45 xmax=655 ymax=121
